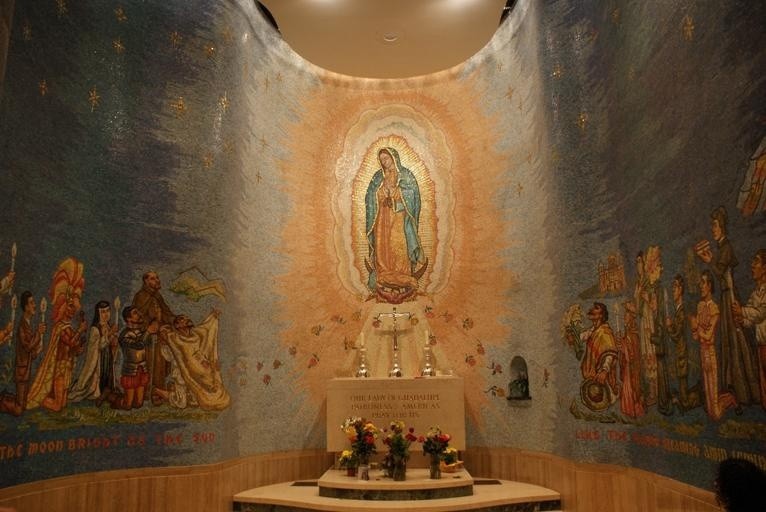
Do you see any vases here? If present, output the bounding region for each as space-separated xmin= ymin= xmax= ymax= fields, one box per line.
xmin=345 ymin=464 xmax=442 ymax=481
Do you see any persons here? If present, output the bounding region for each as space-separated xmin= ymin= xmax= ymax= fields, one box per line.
xmin=663 ymin=274 xmax=705 ymax=416
xmin=632 ymin=243 xmax=667 ymax=407
xmin=150 ymin=305 xmax=231 ymax=411
xmin=0 ymin=291 xmax=46 ymax=417
xmin=693 ymin=206 xmax=761 ymax=408
xmin=0 ymin=321 xmax=13 ymax=347
xmin=1 ymin=272 xmax=14 ymax=294
xmin=613 ymin=301 xmax=647 ymax=416
xmin=67 ymin=297 xmax=124 ymax=403
xmin=684 ymin=269 xmax=744 ymax=419
xmin=642 ymin=285 xmax=677 ymax=415
xmin=95 ymin=306 xmax=158 ymax=409
xmin=557 ymin=300 xmax=621 ymax=412
xmin=132 ymin=271 xmax=177 ymax=399
xmin=363 ymin=147 xmax=426 ymax=276
xmin=730 ymin=248 xmax=766 ymax=405
xmin=22 ymin=257 xmax=88 ymax=411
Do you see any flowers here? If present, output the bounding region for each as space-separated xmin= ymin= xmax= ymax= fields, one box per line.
xmin=339 ymin=416 xmax=457 ymax=468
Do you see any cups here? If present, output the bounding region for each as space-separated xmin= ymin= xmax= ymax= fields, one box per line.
xmin=358 ymin=465 xmax=369 ymax=481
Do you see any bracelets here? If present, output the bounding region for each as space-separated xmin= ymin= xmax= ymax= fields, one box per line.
xmin=2 ymin=338 xmax=6 ymax=344
xmin=3 ymin=328 xmax=8 ymax=335
